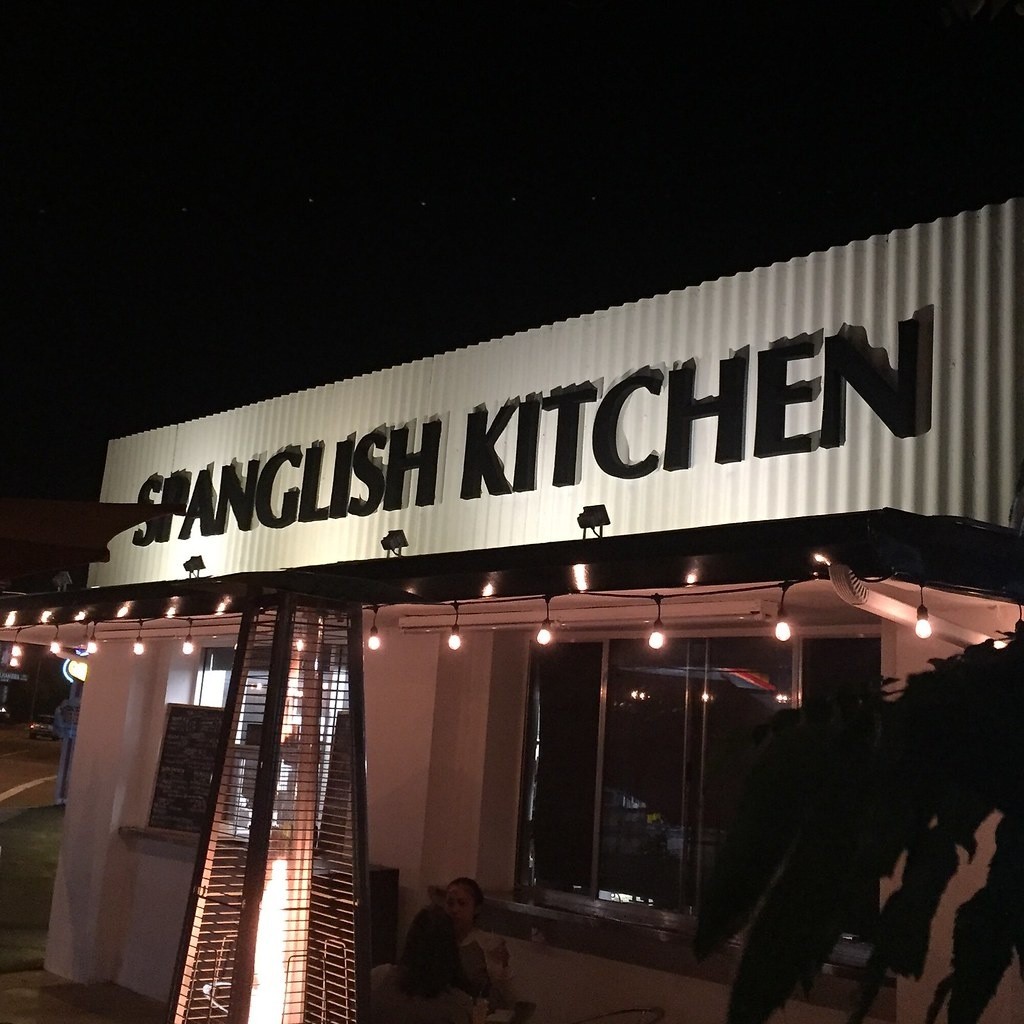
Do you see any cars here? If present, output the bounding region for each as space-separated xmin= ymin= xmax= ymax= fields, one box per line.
xmin=30 ymin=714 xmax=62 ymax=741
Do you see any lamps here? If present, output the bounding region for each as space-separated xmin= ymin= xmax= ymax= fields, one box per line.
xmin=183 ymin=556 xmax=206 ymax=579
xmin=52 ymin=570 xmax=73 ymax=593
xmin=380 ymin=530 xmax=410 ymax=558
xmin=577 ymin=504 xmax=611 ymax=540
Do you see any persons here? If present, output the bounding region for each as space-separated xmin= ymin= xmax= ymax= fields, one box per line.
xmin=367 ymin=906 xmax=488 ymax=1024
xmin=443 ymin=877 xmax=519 ymax=1010
